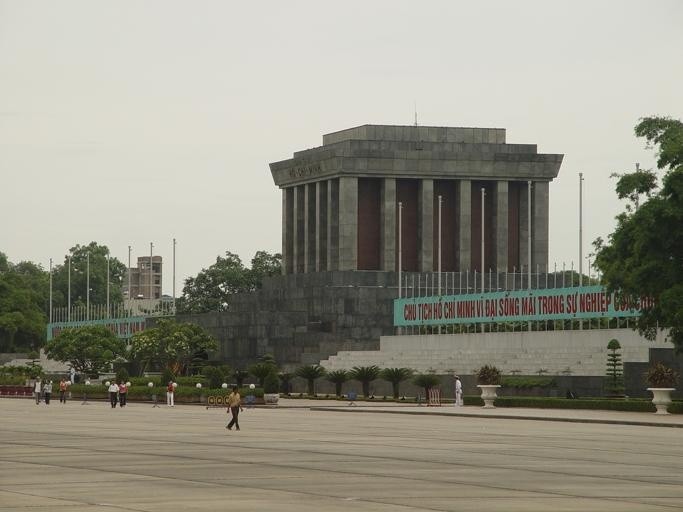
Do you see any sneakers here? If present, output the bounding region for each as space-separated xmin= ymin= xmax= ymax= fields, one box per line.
xmin=226 ymin=425 xmax=240 ymax=431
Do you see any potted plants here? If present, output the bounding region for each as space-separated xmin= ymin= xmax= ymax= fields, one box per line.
xmin=261 ymin=373 xmax=279 ymax=404
xmin=474 ymin=362 xmax=501 ymax=408
xmin=642 ymin=362 xmax=679 ymax=414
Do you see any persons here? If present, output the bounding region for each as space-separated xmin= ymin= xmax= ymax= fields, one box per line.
xmin=454 ymin=375 xmax=463 ymax=406
xmin=166 ymin=382 xmax=175 ymax=407
xmin=33 ymin=376 xmax=43 ymax=405
xmin=226 ymin=386 xmax=243 ymax=430
xmin=108 ymin=380 xmax=120 ymax=408
xmin=59 ymin=377 xmax=66 ymax=403
xmin=118 ymin=380 xmax=128 ymax=407
xmin=43 ymin=380 xmax=52 ymax=406
